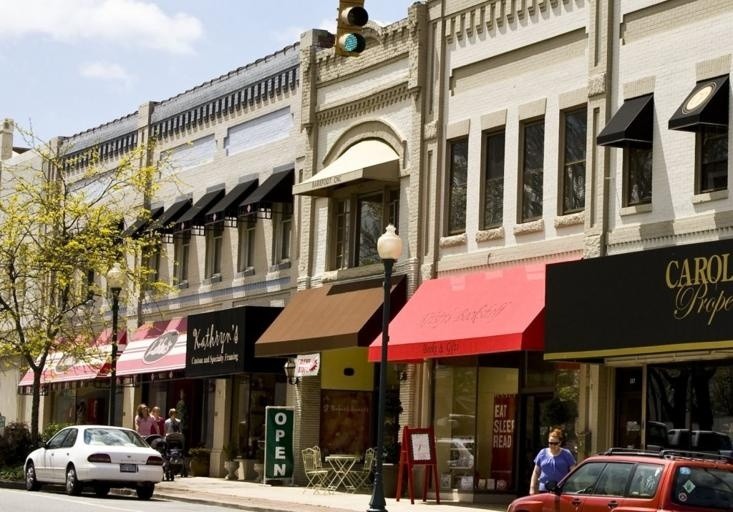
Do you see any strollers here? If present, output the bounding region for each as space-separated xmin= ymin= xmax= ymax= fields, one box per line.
xmin=145 ymin=431 xmax=188 ymax=480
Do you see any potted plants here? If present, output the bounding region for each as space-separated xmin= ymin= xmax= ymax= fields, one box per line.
xmin=188 ymin=448 xmax=209 ymax=477
xmin=223 ymin=439 xmax=239 ymax=481
xmin=252 ymin=447 xmax=264 ymax=483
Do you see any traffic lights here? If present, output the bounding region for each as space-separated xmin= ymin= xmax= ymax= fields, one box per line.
xmin=336 ymin=1 xmax=367 ymax=56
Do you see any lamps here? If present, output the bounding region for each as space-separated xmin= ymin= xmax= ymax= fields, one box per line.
xmin=392 ymin=363 xmax=407 ymax=380
xmin=284 ymin=359 xmax=299 ymax=386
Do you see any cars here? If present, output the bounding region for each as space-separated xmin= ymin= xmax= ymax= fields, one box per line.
xmin=507 ymin=447 xmax=733 ymax=512
xmin=434 ymin=416 xmax=474 ymax=469
xmin=644 ymin=421 xmax=733 ymax=456
xmin=20 ymin=424 xmax=163 ymax=498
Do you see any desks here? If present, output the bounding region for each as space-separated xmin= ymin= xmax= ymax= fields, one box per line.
xmin=233 ymin=458 xmax=255 ymax=481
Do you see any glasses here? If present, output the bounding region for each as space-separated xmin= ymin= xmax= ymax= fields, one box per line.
xmin=549 ymin=442 xmax=559 ymax=445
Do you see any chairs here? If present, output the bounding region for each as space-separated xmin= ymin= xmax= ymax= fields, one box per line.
xmin=301 ymin=446 xmax=377 ymax=494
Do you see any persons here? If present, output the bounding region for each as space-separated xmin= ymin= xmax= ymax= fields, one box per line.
xmin=133 ymin=405 xmax=185 ymax=482
xmin=527 ymin=427 xmax=577 ymax=495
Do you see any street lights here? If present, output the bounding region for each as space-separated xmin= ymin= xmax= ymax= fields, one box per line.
xmin=105 ymin=262 xmax=124 ymax=425
xmin=365 ymin=223 xmax=402 ymax=511
